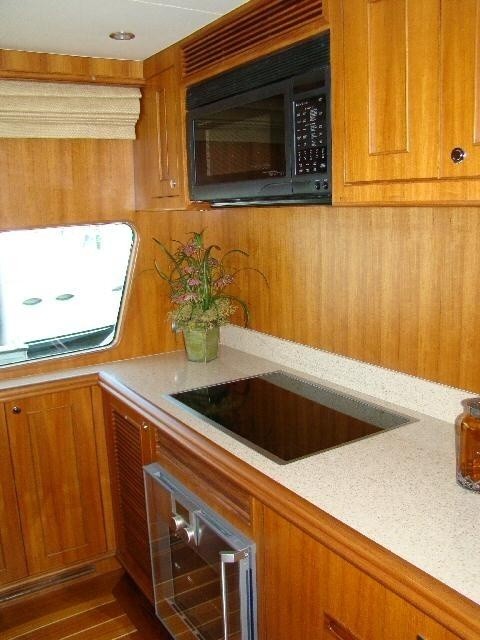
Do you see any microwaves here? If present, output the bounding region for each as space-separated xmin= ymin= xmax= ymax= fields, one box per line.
xmin=181 ymin=64 xmax=334 ymax=210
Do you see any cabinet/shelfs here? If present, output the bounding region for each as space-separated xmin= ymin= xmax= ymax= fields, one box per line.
xmin=0 ymin=372 xmax=117 ymax=608
xmin=133 ymin=65 xmax=183 ymax=212
xmin=100 ymin=388 xmax=154 ymax=607
xmin=330 ymin=0 xmax=480 ymax=210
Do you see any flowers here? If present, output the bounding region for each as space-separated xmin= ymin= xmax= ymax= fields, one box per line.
xmin=132 ymin=225 xmax=271 ymax=337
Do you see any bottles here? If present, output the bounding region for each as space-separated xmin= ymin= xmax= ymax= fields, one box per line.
xmin=453 ymin=396 xmax=480 ymax=496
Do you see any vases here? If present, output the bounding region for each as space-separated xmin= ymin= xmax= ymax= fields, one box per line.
xmin=182 ymin=321 xmax=220 ymax=363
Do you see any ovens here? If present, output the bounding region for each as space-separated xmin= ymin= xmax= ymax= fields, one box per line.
xmin=140 ymin=459 xmax=259 ymax=638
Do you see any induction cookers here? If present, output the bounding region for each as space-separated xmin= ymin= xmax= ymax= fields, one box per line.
xmin=161 ymin=368 xmax=422 ymax=467
